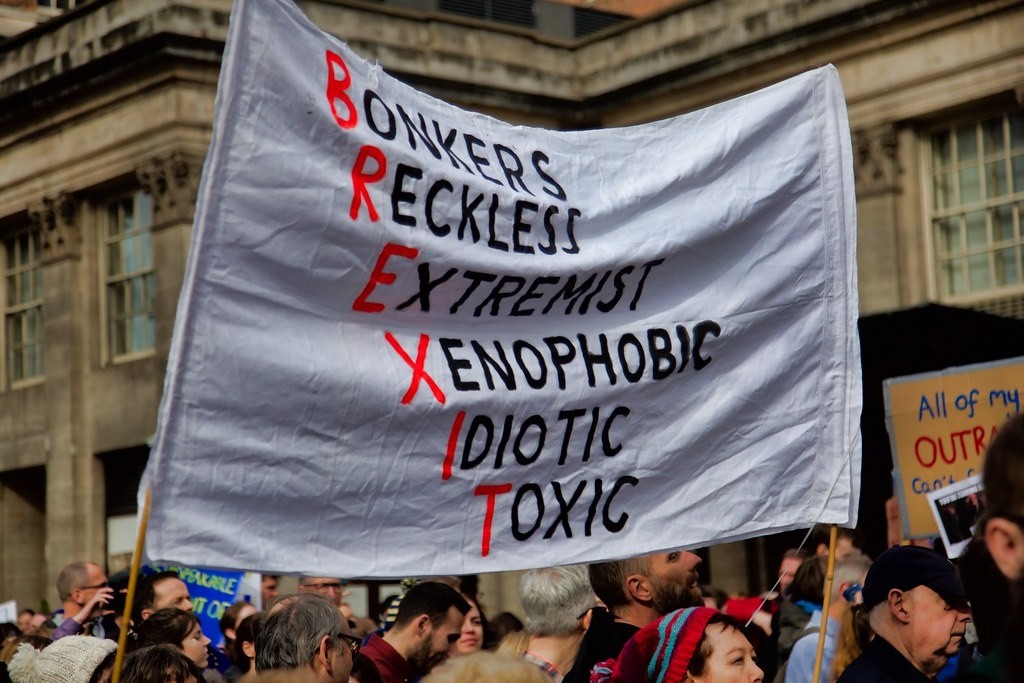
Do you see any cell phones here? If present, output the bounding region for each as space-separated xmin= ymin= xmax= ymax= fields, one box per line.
xmin=842 ymin=582 xmax=864 ymax=602
xmin=104 ymin=593 xmax=127 ymax=611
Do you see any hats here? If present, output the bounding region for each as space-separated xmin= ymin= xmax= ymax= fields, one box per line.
xmin=7 ymin=635 xmax=118 ymax=683
xmin=862 ymin=544 xmax=969 ymax=614
xmin=589 ymin=606 xmax=722 ymax=683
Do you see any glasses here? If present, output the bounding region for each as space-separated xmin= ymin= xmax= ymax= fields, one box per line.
xmin=68 ymin=581 xmax=110 ymax=597
xmin=304 ymin=582 xmax=346 ymax=594
xmin=328 ymin=630 xmax=362 ymax=660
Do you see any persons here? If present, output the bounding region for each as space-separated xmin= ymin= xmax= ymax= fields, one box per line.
xmin=0 ymin=412 xmax=1024 ymax=683
